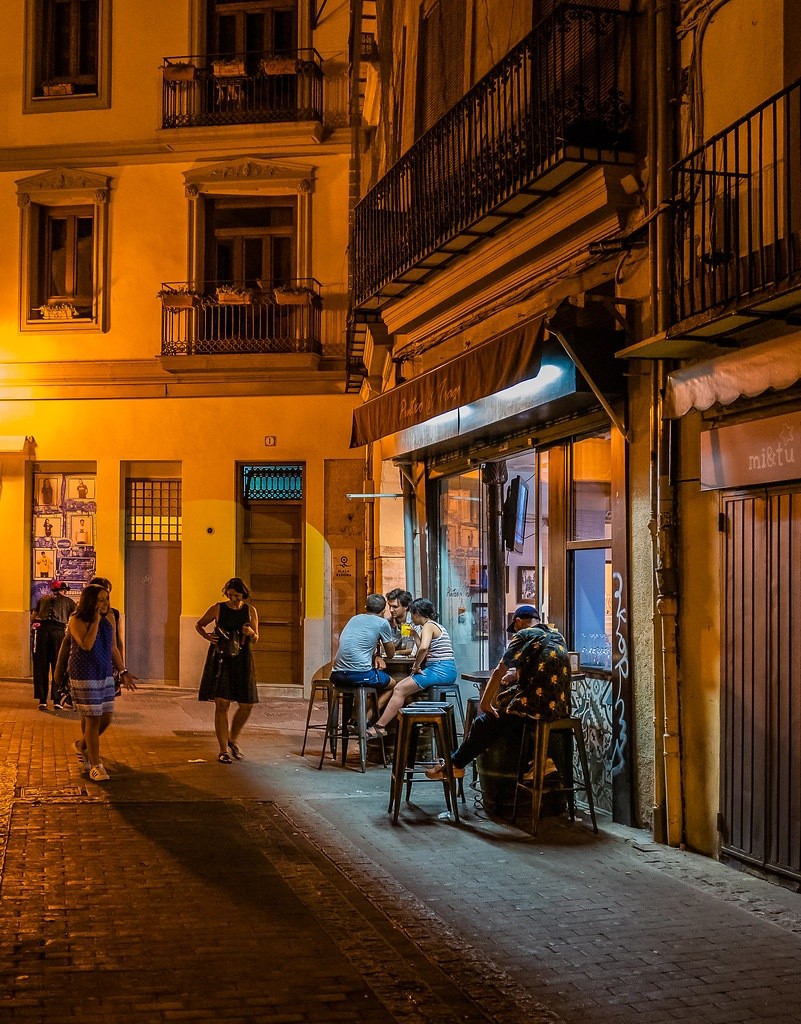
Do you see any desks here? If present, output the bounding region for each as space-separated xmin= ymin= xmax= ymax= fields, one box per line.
xmin=340 ymin=654 xmax=419 ymax=768
xmin=461 ymin=670 xmax=585 ymax=701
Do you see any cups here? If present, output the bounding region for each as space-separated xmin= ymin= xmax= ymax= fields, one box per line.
xmin=401 ymin=622 xmax=410 ymax=637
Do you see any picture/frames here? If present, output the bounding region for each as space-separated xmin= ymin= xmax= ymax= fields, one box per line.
xmin=517 ymin=566 xmax=544 ymax=604
xmin=507 ymin=613 xmax=545 ymax=640
xmin=471 ymin=603 xmax=490 ymax=641
xmin=478 ymin=566 xmax=509 ymax=593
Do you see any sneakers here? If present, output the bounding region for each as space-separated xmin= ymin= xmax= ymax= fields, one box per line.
xmin=522 ymin=755 xmax=559 ymax=783
xmin=88 ymin=759 xmax=110 ymax=781
xmin=424 ymin=761 xmax=465 ymax=779
xmin=71 ymin=740 xmax=92 ymax=773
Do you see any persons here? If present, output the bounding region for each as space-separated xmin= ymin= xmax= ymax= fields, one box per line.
xmin=43 ymin=519 xmax=53 ymax=537
xmin=41 ymin=479 xmax=53 ymax=504
xmin=330 ymin=594 xmax=397 ymax=732
xmin=77 ymin=479 xmax=88 ymax=498
xmin=68 ymin=584 xmax=138 ymax=781
xmin=32 ymin=581 xmax=78 ymax=708
xmin=379 ymin=588 xmax=421 ymax=656
xmin=75 ymin=519 xmax=88 ymax=544
xmin=349 ymin=598 xmax=456 ymax=739
xmin=54 ymin=577 xmax=124 ymax=772
xmin=37 ymin=552 xmax=53 ymax=577
xmin=425 ymin=606 xmax=572 ymax=781
xmin=196 ymin=578 xmax=258 ymax=763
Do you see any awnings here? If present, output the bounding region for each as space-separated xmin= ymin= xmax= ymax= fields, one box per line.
xmin=349 ymin=294 xmax=567 ymax=449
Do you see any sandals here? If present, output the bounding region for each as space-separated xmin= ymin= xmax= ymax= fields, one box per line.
xmin=366 ymin=723 xmax=388 ymax=740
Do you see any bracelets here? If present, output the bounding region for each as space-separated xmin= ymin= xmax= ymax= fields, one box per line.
xmin=411 ymin=667 xmax=421 ymax=671
xmin=120 ymin=669 xmax=127 ymax=675
xmin=252 ymin=633 xmax=256 ymax=637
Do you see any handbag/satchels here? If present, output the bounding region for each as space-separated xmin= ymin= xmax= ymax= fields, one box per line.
xmin=210 ymin=624 xmax=241 ymax=659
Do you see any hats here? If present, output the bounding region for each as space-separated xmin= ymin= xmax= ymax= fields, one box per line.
xmin=51 ymin=580 xmax=71 ymax=592
xmin=506 ymin=606 xmax=540 ymax=634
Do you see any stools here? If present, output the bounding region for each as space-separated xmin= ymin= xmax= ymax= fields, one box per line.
xmin=466 ymin=695 xmax=487 ymax=787
xmin=406 ymin=701 xmax=466 ymax=805
xmin=387 ymin=708 xmax=461 ymax=827
xmin=412 ymin=684 xmax=466 ymax=768
xmin=300 ymin=679 xmax=338 ymax=756
xmin=318 ymin=688 xmax=387 ymax=773
xmin=511 ymin=716 xmax=599 ymax=837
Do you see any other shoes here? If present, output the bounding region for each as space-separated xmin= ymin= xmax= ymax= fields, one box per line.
xmin=217 ymin=751 xmax=233 ymax=764
xmin=347 ymin=717 xmax=358 ymax=735
xmin=38 ymin=702 xmax=48 ymax=711
xmin=227 ymin=738 xmax=244 ymax=760
xmin=52 ymin=703 xmax=64 ymax=710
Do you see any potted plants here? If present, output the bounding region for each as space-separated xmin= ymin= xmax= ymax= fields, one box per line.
xmin=214 ymin=285 xmax=257 ymax=304
xmin=157 ymin=288 xmax=201 ymax=309
xmin=211 ymin=57 xmax=249 ymax=78
xmin=258 ymin=53 xmax=299 ymax=75
xmin=159 ymin=61 xmax=197 ymax=81
xmin=40 ymin=79 xmax=75 ymax=95
xmin=39 ymin=303 xmax=79 ymax=319
xmin=271 ymin=286 xmax=315 ymax=305
